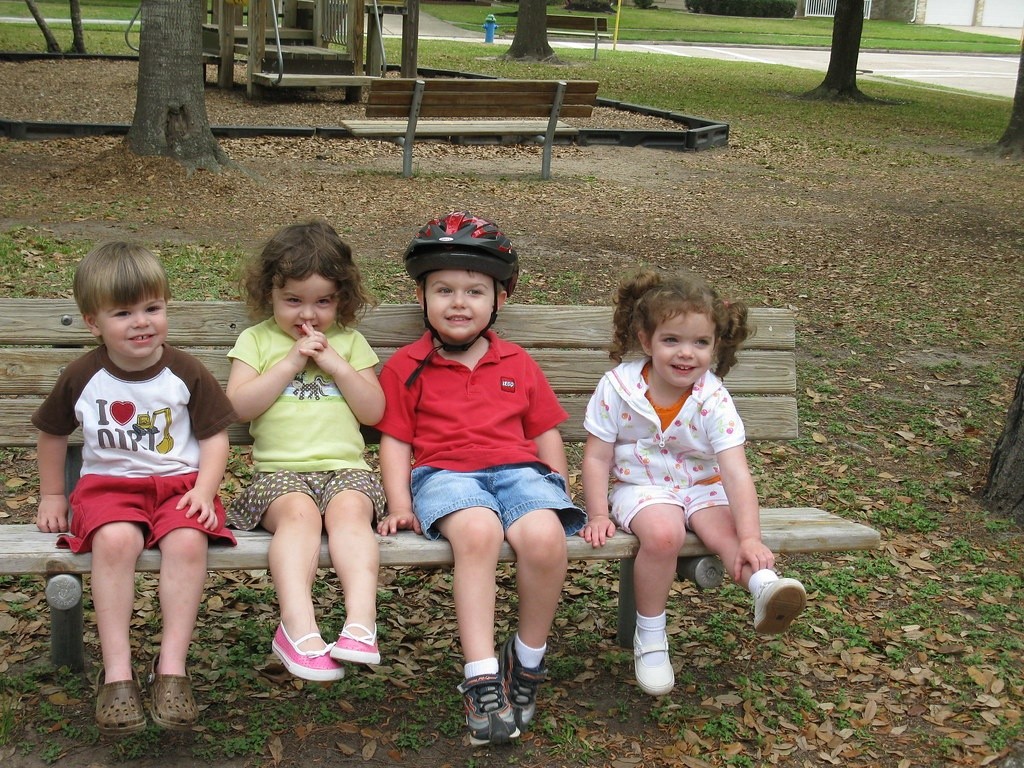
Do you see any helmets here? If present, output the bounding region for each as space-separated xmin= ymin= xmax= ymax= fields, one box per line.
xmin=402 ymin=212 xmax=519 ymax=297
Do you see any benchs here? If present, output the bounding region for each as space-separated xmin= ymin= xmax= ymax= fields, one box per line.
xmin=546 ymin=14 xmax=613 ymax=61
xmin=0 ymin=297 xmax=880 ymax=672
xmin=340 ymin=78 xmax=599 ymax=180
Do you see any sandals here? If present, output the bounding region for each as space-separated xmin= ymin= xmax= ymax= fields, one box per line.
xmin=94 ymin=665 xmax=146 ymax=735
xmin=146 ymin=651 xmax=199 ymax=731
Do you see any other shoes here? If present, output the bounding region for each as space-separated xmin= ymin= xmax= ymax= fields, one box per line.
xmin=271 ymin=619 xmax=345 ymax=681
xmin=755 ymin=578 xmax=807 ymax=635
xmin=633 ymin=628 xmax=675 ymax=696
xmin=329 ymin=617 xmax=382 ymax=664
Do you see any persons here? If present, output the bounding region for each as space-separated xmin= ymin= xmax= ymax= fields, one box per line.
xmin=34 ymin=242 xmax=231 ymax=739
xmin=579 ymin=273 xmax=807 ymax=695
xmin=226 ymin=222 xmax=386 ymax=684
xmin=375 ymin=214 xmax=572 ymax=745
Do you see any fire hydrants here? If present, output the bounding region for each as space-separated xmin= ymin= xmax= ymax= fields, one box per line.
xmin=482 ymin=13 xmax=499 ymax=43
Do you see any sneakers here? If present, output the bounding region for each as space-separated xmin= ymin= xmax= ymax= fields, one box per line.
xmin=500 ymin=635 xmax=548 ymax=729
xmin=456 ymin=672 xmax=521 ymax=747
